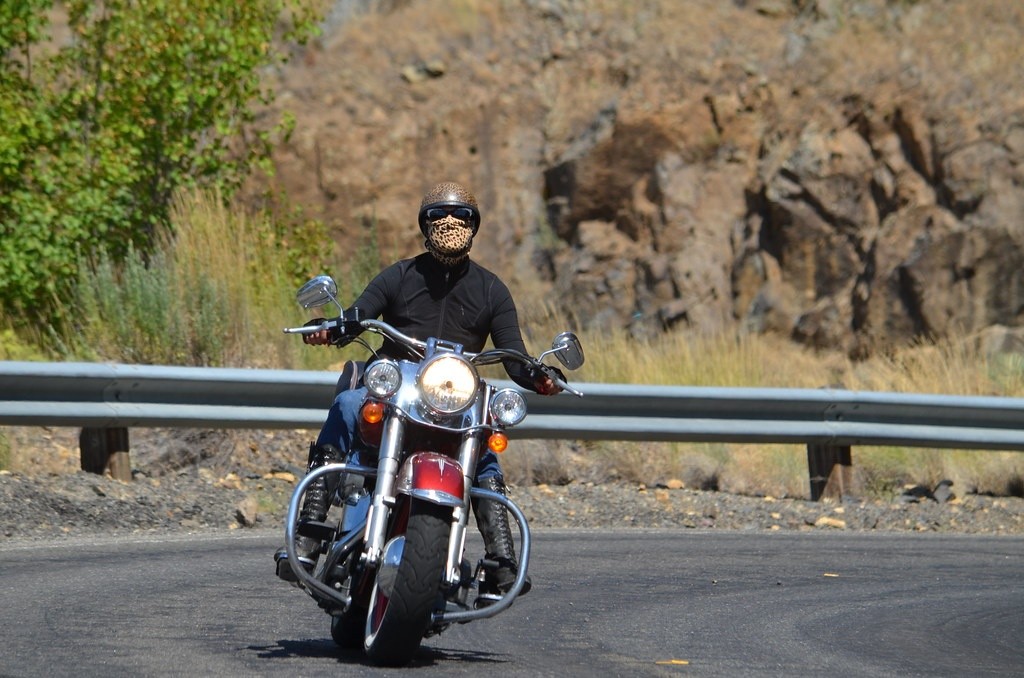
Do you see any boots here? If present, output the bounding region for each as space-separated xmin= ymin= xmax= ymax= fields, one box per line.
xmin=470 ymin=478 xmax=532 ymax=597
xmin=273 ymin=441 xmax=345 ymax=581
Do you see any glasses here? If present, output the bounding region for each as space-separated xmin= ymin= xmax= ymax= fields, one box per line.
xmin=428 ymin=208 xmax=473 ymax=223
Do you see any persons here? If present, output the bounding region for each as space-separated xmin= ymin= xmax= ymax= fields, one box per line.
xmin=272 ymin=181 xmax=569 ymax=595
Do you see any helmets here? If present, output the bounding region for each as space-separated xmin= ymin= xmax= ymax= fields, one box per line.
xmin=418 ymin=181 xmax=482 ymax=238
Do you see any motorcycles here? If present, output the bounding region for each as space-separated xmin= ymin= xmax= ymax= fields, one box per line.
xmin=275 ymin=274 xmax=585 ymax=660
